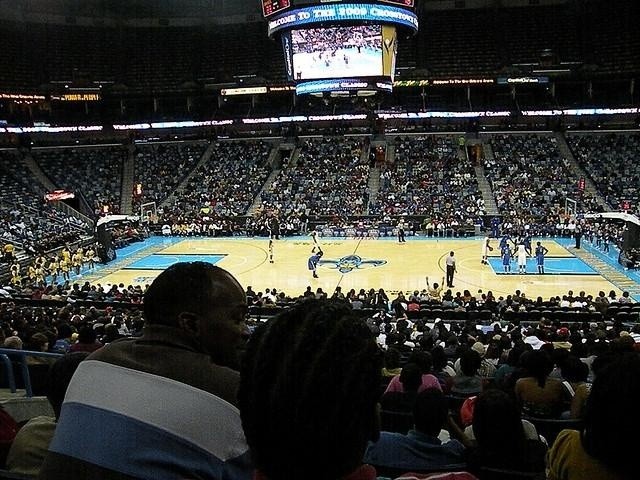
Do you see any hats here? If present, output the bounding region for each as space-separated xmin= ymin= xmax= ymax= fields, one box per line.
xmin=559 ymin=328 xmax=569 ymax=336
xmin=471 ymin=342 xmax=484 ymax=354
xmin=461 ymin=397 xmax=478 ymax=427
xmin=493 ymin=335 xmax=501 ymax=340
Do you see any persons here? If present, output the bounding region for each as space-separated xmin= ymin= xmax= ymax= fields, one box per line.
xmin=0 ymin=115 xmax=640 ymax=480
xmin=292 ymin=26 xmax=382 ymax=69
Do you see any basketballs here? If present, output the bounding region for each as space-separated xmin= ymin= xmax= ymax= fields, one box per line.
xmin=489 ymin=247 xmax=493 ymax=251
